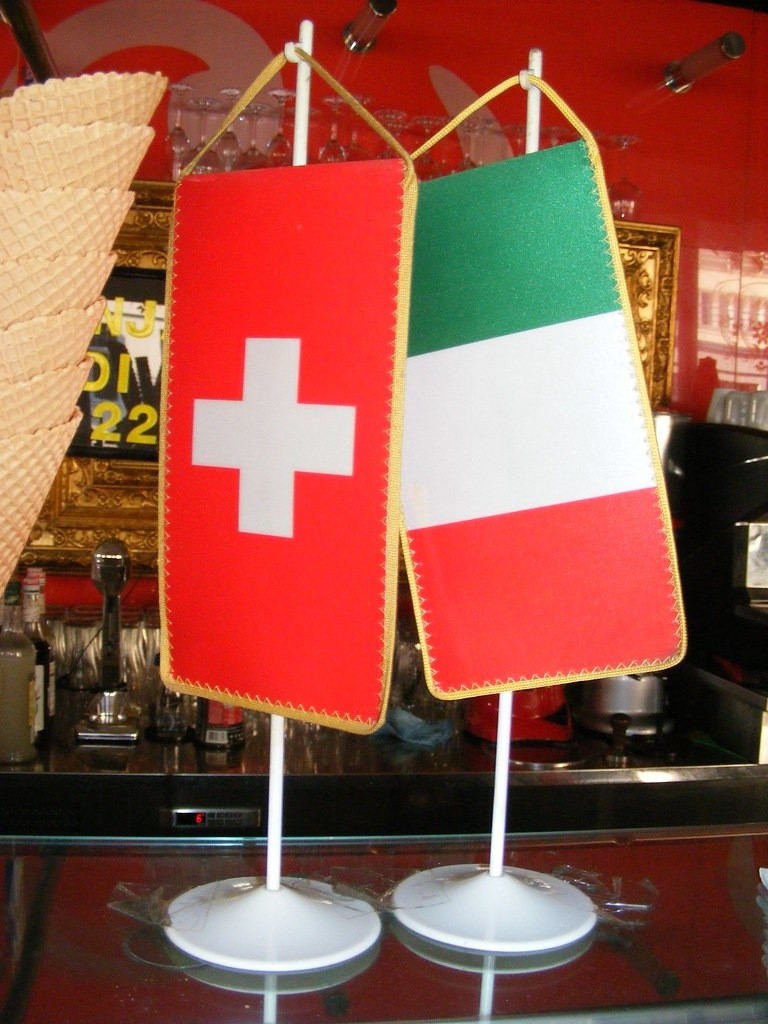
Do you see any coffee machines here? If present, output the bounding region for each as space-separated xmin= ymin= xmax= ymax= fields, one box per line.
xmin=581 ymin=400 xmax=693 ymax=744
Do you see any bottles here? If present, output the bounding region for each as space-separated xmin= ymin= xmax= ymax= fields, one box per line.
xmin=0 ymin=565 xmax=60 ymax=768
